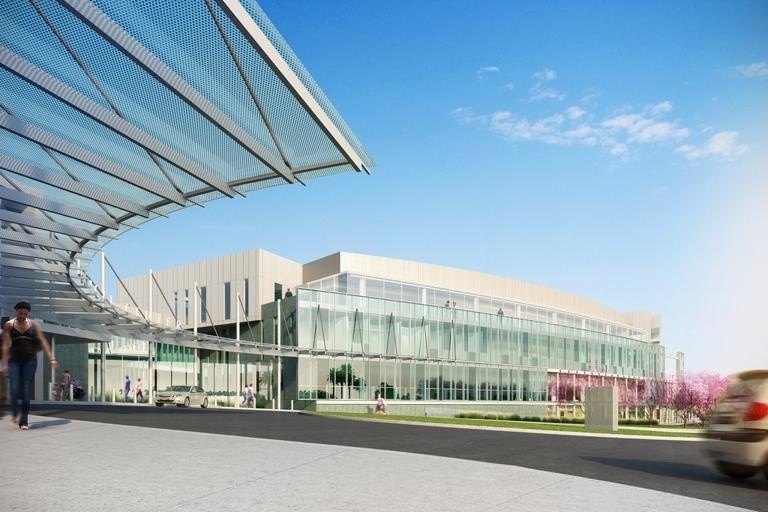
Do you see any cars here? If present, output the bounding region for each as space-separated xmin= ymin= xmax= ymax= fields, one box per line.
xmin=699 ymin=367 xmax=768 ymax=488
xmin=155 ymin=385 xmax=209 ymax=411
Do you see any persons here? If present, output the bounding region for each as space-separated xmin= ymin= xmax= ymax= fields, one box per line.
xmin=285 ymin=288 xmax=293 ymax=298
xmin=123 ymin=375 xmax=131 ymax=401
xmin=451 ymin=302 xmax=458 ymax=310
xmin=52 ymin=367 xmax=82 ymax=401
xmin=376 ymin=393 xmax=385 ymax=415
xmin=241 ymin=384 xmax=248 ymax=405
xmin=445 ymin=298 xmax=449 ymax=309
xmin=136 ymin=377 xmax=144 ymax=402
xmin=247 ymin=383 xmax=254 ymax=408
xmin=0 ymin=300 xmax=61 ymax=430
xmin=497 ymin=308 xmax=504 ymax=315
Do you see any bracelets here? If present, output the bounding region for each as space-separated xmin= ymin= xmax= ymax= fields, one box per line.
xmin=48 ymin=356 xmax=57 ymax=360
